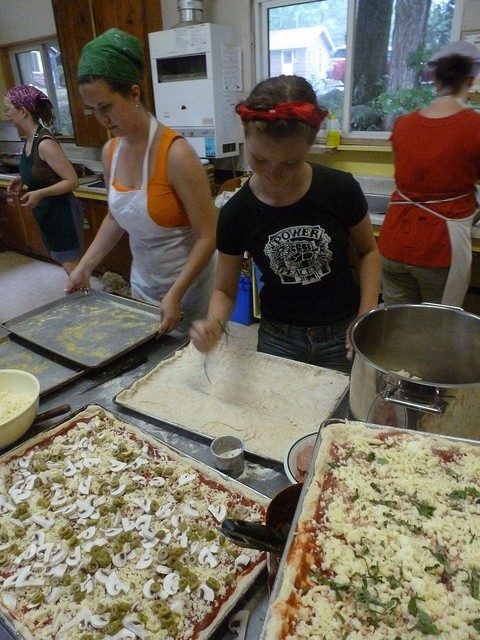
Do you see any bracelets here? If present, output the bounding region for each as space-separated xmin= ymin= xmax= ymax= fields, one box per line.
xmin=205 ymin=312 xmax=236 ymax=347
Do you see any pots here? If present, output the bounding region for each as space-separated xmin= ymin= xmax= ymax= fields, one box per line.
xmin=265 ymin=483 xmax=305 ymax=596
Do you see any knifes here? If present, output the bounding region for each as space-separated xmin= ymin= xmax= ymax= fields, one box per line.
xmin=80 ymin=355 xmax=149 ymax=394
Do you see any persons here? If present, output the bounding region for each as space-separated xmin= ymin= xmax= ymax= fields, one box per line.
xmin=377 ymin=41 xmax=480 ymax=307
xmin=189 ymin=75 xmax=381 ymax=375
xmin=5 ymin=85 xmax=84 ymax=275
xmin=62 ymin=26 xmax=217 ymax=338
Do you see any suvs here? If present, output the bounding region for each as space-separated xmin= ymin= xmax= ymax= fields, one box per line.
xmin=326 ymin=47 xmax=425 ymax=84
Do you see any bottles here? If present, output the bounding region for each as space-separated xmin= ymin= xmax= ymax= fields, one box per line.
xmin=326 ymin=111 xmax=340 ymax=146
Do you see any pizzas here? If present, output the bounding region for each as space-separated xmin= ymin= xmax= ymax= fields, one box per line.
xmin=0 ymin=408 xmax=273 ymax=640
xmin=260 ymin=417 xmax=480 ymax=640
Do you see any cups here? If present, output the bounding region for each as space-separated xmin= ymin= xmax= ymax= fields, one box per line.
xmin=210 ymin=436 xmax=244 ymax=480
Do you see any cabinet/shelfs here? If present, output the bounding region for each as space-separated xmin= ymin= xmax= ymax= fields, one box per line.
xmin=51 ymin=0 xmax=164 ymax=146
xmin=449 ymin=0 xmax=480 ymax=101
xmin=0 ymin=181 xmax=134 ymax=283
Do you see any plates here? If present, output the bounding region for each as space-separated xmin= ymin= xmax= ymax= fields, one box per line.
xmin=365 ymin=392 xmax=407 ymax=430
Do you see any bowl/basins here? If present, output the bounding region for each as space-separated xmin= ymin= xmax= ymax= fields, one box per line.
xmin=0 ymin=369 xmax=39 ymax=451
xmin=284 ymin=431 xmax=320 ymax=486
